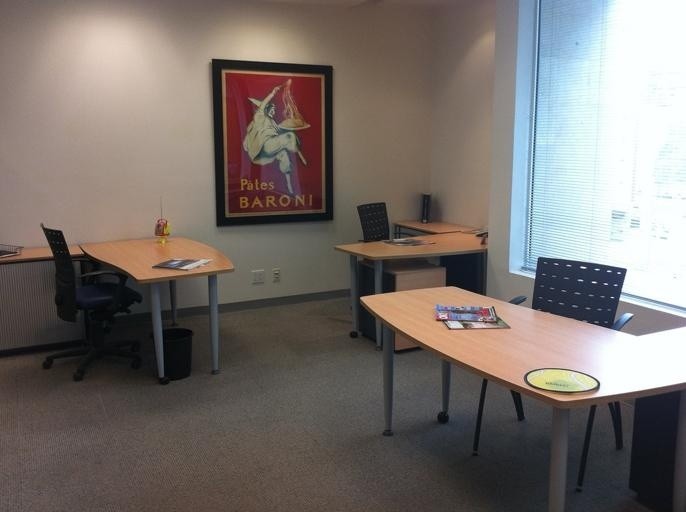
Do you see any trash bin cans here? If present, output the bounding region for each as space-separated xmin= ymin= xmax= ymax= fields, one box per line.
xmin=161 ymin=324 xmax=195 ymax=379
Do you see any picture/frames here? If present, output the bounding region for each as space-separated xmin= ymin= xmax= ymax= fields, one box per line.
xmin=211 ymin=59 xmax=335 ymax=228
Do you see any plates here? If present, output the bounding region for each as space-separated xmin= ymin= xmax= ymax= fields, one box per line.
xmin=278 ymin=122 xmax=311 ymax=131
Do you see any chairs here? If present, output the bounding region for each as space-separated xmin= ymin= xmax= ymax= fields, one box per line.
xmin=473 ymin=257 xmax=635 ymax=491
xmin=38 ymin=220 xmax=144 ymax=381
xmin=352 ymin=201 xmax=417 ymax=242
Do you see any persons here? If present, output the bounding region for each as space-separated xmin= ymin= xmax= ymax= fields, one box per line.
xmin=241 ymin=85 xmax=313 ymax=199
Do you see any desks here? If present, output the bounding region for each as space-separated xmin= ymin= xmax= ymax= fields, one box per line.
xmin=359 ymin=286 xmax=686 ymax=512
xmin=334 ymin=221 xmax=490 ymax=349
xmin=81 ymin=233 xmax=235 ymax=384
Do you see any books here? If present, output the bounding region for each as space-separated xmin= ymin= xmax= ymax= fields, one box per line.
xmin=384 ymin=237 xmax=435 ymax=245
xmin=151 ymin=257 xmax=214 ymax=272
xmin=434 ymin=303 xmax=512 ymax=331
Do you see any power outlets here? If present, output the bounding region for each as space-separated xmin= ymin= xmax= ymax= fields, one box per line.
xmin=251 ymin=270 xmax=264 ymax=283
xmin=272 ymin=268 xmax=280 ymax=280
